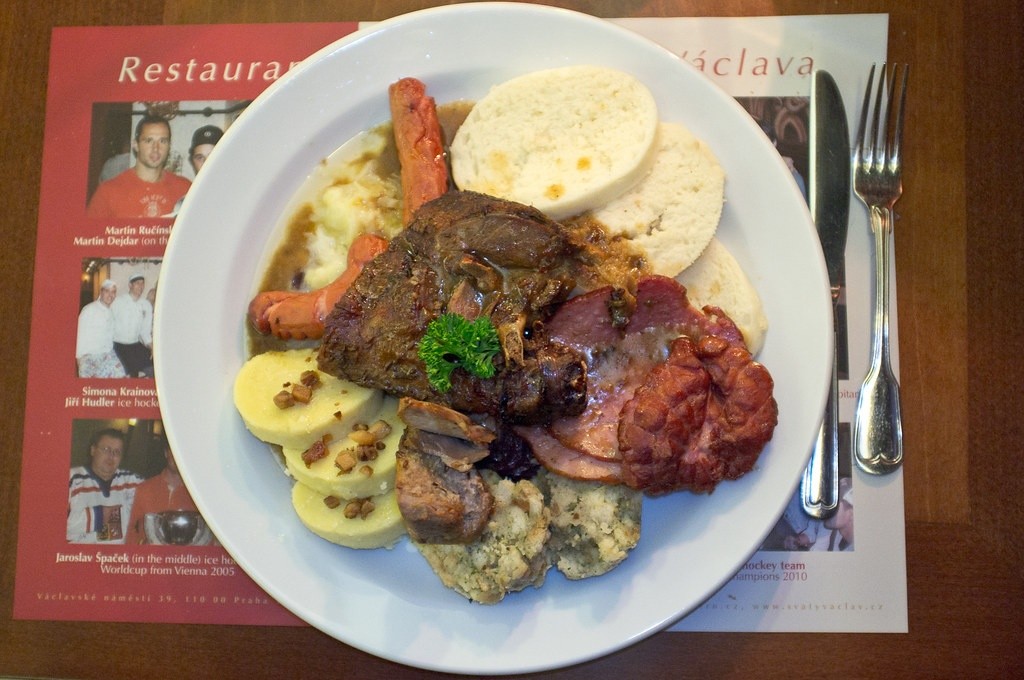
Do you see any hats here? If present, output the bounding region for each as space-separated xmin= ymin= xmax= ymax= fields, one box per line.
xmin=95 ymin=445 xmax=123 ymax=456
xmin=101 ymin=279 xmax=116 ymax=289
xmin=188 ymin=125 xmax=224 ymax=151
xmin=129 ymin=272 xmax=144 ymax=281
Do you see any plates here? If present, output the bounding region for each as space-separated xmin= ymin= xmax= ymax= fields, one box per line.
xmin=153 ymin=3 xmax=835 ymax=674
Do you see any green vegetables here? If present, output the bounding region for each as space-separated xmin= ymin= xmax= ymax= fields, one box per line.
xmin=417 ymin=312 xmax=502 ymax=393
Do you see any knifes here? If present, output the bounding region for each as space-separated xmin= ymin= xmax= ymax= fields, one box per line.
xmin=801 ymin=68 xmax=853 ymax=520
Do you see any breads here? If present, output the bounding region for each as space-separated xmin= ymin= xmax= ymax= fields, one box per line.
xmin=448 ymin=66 xmax=764 ymax=362
xmin=232 ymin=348 xmax=412 ymax=550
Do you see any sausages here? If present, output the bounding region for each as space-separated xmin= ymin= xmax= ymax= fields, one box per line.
xmin=389 ymin=77 xmax=450 ymax=229
xmin=245 ymin=236 xmax=391 ymax=340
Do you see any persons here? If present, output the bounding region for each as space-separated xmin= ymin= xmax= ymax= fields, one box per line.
xmin=770 ymin=470 xmax=854 ymax=552
xmin=75 ymin=271 xmax=158 ymax=379
xmin=86 ymin=114 xmax=224 ymax=218
xmin=65 ymin=427 xmax=221 ymax=547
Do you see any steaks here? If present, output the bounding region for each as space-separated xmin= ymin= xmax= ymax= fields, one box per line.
xmin=314 ymin=190 xmax=589 ymax=422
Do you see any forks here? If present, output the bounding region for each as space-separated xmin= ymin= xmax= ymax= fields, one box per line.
xmin=854 ymin=61 xmax=911 ymax=477
